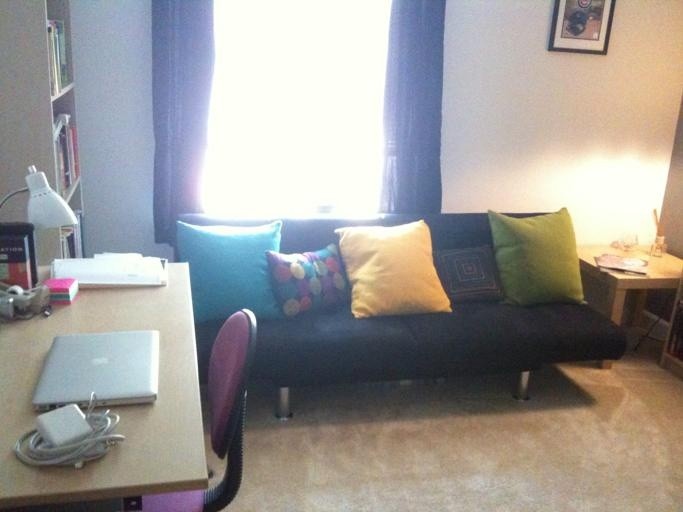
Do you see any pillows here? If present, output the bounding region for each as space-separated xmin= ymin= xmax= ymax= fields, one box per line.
xmin=336 ymin=218 xmax=453 ymax=318
xmin=266 ymin=243 xmax=347 ymax=320
xmin=177 ymin=220 xmax=282 ymax=317
xmin=434 ymin=243 xmax=503 ymax=305
xmin=489 ymin=209 xmax=589 ymax=306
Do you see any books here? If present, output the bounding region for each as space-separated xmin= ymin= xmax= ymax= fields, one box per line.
xmin=49 ymin=251 xmax=171 ymax=290
xmin=43 ymin=18 xmax=85 ymax=258
xmin=592 ymin=251 xmax=652 ymax=276
xmin=0 ymin=219 xmax=37 ymax=292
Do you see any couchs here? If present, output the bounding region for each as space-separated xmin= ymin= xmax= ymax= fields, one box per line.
xmin=172 ymin=211 xmax=627 ymax=422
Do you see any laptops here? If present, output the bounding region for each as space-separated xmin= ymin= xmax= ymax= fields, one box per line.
xmin=31 ymin=330 xmax=161 ymax=412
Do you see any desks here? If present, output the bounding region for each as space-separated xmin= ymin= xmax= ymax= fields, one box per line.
xmin=0 ymin=260 xmax=209 ymax=512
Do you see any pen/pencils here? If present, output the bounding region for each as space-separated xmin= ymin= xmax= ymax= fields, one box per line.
xmin=653 ymin=208 xmax=664 ymax=237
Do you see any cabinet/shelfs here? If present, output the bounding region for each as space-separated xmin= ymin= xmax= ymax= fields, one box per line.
xmin=0 ymin=1 xmax=95 ymax=260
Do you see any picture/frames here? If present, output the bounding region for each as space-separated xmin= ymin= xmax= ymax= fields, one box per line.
xmin=545 ymin=0 xmax=617 ymax=55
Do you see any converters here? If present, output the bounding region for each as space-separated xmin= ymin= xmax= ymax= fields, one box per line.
xmin=35 ymin=403 xmax=92 ymax=446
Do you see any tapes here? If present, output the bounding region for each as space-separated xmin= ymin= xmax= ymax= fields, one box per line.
xmin=7 ymin=284 xmax=24 ymax=296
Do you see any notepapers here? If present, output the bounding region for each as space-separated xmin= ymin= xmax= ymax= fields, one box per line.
xmin=43 ymin=278 xmax=81 ymax=304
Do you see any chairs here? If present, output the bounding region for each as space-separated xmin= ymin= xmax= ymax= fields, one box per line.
xmin=140 ymin=308 xmax=259 ymax=512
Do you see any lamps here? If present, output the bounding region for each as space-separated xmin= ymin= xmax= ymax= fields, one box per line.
xmin=1 ymin=165 xmax=84 ymax=232
